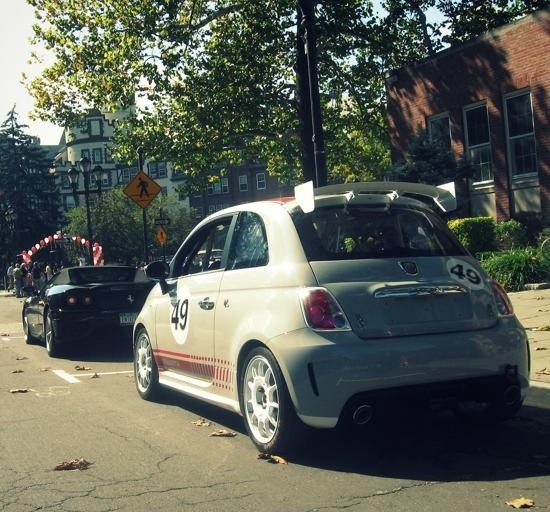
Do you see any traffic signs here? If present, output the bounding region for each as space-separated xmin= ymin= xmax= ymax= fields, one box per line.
xmin=156 ymin=219 xmax=171 ymax=224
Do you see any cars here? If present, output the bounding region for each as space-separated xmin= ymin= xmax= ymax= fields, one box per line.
xmin=128 ymin=179 xmax=531 ymax=457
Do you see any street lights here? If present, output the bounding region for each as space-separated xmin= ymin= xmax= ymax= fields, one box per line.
xmin=66 ymin=154 xmax=105 ymax=267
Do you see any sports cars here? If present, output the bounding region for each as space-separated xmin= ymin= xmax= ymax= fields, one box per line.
xmin=21 ymin=264 xmax=155 ymax=357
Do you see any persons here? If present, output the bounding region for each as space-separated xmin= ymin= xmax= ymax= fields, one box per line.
xmin=5 ymin=260 xmax=68 ymax=298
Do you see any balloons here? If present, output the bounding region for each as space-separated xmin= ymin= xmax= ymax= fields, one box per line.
xmin=22 ymin=232 xmax=105 ymax=267
